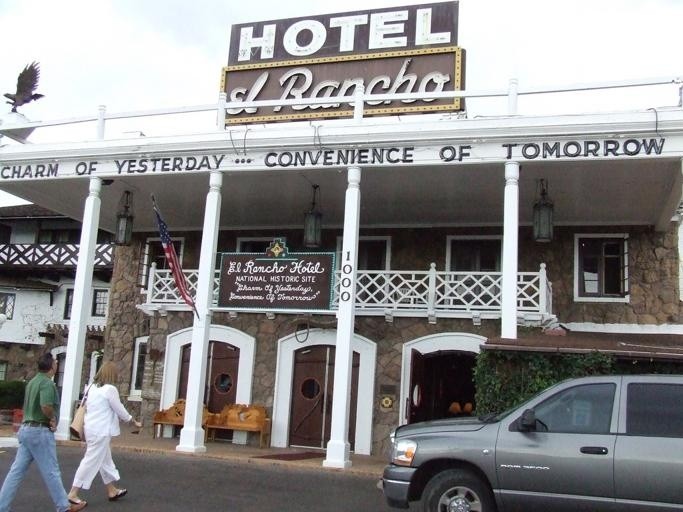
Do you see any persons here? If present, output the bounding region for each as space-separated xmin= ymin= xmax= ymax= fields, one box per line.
xmin=0 ymin=353 xmax=88 ymax=512
xmin=68 ymin=361 xmax=143 ymax=505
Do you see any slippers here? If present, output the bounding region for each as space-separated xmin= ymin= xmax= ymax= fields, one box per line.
xmin=68 ymin=497 xmax=88 ymax=504
xmin=108 ymin=488 xmax=128 ymax=500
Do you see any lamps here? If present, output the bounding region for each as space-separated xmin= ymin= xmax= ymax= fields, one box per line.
xmin=303 ymin=184 xmax=323 ymax=249
xmin=531 ymin=175 xmax=555 ymax=243
xmin=116 ymin=189 xmax=134 ymax=246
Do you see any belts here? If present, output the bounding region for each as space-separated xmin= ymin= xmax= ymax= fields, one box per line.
xmin=30 ymin=421 xmax=49 ymax=427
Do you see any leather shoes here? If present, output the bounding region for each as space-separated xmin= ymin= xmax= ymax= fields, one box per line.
xmin=69 ymin=501 xmax=87 ymax=512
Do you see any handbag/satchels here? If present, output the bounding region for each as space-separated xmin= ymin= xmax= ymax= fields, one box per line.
xmin=70 ymin=406 xmax=85 ymax=440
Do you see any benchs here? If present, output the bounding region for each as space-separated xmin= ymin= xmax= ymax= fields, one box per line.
xmin=148 ymin=399 xmax=273 ymax=450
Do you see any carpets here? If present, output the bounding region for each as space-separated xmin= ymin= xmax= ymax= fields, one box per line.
xmin=247 ymin=451 xmax=326 ymax=461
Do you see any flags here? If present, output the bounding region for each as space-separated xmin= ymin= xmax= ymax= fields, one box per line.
xmin=151 ymin=198 xmax=195 ymax=307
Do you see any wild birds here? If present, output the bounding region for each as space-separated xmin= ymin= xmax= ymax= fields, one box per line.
xmin=3 ymin=61 xmax=45 ymax=113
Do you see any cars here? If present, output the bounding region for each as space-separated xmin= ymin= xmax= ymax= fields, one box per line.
xmin=382 ymin=374 xmax=682 ymax=512
